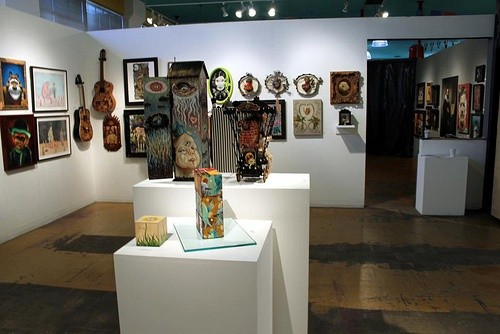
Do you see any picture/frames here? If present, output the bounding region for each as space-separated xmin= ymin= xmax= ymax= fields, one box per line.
xmin=264 ymin=100 xmax=287 ymax=139
xmin=123 ymin=110 xmax=146 ymax=158
xmin=35 ymin=115 xmax=71 ymax=160
xmin=414 ymin=65 xmax=485 ymax=139
xmin=207 ymin=67 xmax=232 ymax=104
xmin=123 ymin=56 xmax=158 ymax=105
xmin=0 ymin=57 xmax=28 ymax=110
xmin=31 ymin=65 xmax=69 ymax=112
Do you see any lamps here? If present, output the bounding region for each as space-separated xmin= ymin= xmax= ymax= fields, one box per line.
xmin=146 ymin=10 xmax=169 ymax=27
xmin=248 ymin=1 xmax=256 ymax=17
xmin=376 ymin=8 xmax=389 ymax=18
xmin=342 ymin=0 xmax=351 ymax=12
xmin=236 ymin=1 xmax=247 ymax=18
xmin=268 ymin=1 xmax=277 ymax=17
xmin=221 ymin=2 xmax=228 ymax=17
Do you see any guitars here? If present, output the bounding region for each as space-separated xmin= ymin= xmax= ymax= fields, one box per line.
xmin=73 ymin=74 xmax=93 ymax=144
xmin=92 ymin=48 xmax=117 ymax=114
